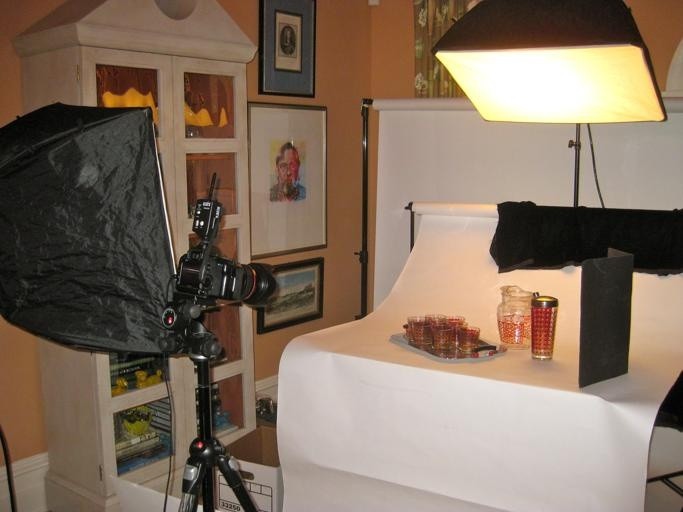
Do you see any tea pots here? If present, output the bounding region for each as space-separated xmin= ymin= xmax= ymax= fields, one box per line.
xmin=497 ymin=285 xmax=539 ymax=349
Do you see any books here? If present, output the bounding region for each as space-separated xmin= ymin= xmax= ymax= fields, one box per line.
xmin=109 ymin=357 xmax=155 ymax=388
xmin=146 ymin=383 xmax=239 ymax=438
xmin=115 ymin=430 xmax=171 ymax=475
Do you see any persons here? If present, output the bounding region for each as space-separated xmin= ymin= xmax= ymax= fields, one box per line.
xmin=270 ymin=142 xmax=306 ymax=201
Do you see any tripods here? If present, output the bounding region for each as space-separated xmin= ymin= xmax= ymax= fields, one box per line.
xmin=179 ymin=358 xmax=257 ymax=512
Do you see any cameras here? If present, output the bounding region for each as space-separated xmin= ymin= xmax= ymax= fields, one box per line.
xmin=175 ymin=198 xmax=277 ymax=309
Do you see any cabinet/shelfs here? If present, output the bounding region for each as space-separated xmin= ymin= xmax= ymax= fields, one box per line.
xmin=13 ymin=0 xmax=255 ymax=512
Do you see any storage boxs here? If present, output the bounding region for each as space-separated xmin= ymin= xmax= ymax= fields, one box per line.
xmin=215 ymin=425 xmax=283 ymax=512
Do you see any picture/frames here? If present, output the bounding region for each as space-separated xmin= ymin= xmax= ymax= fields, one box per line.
xmin=256 ymin=0 xmax=315 ymax=97
xmin=257 ymin=257 xmax=324 ymax=334
xmin=246 ymin=102 xmax=327 ymax=261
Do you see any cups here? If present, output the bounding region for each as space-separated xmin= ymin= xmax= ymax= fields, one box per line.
xmin=406 ymin=313 xmax=481 ymax=354
xmin=530 ymin=296 xmax=559 ymax=361
